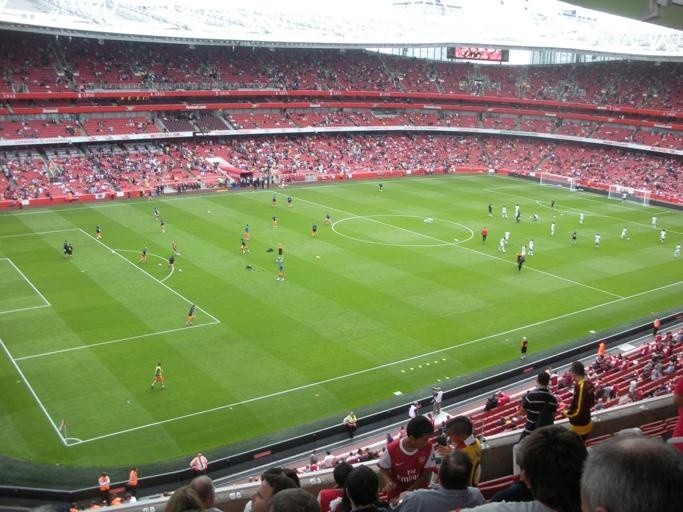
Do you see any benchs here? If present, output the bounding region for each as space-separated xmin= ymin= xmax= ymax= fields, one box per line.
xmin=304 ymin=330 xmax=682 ymax=512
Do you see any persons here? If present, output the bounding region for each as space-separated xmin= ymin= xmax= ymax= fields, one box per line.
xmin=0 ymin=25 xmax=682 ymax=512
